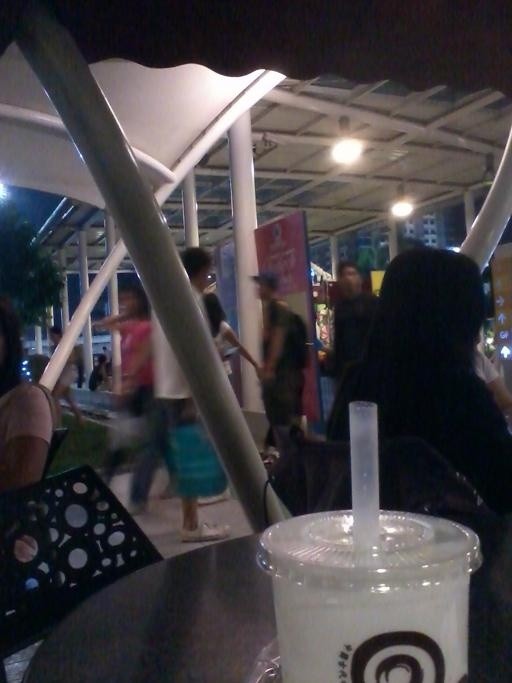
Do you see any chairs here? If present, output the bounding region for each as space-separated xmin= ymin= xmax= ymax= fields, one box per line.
xmin=302 ymin=436 xmax=500 ymax=516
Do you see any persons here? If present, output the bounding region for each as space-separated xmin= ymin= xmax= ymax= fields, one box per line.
xmin=0 ymin=200 xmax=511 ymax=648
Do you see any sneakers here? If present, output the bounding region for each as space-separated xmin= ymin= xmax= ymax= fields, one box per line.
xmin=259 ymin=449 xmax=280 ymax=464
xmin=159 ymin=486 xmax=176 ymax=499
xmin=197 ymin=488 xmax=229 ymax=504
xmin=179 ymin=520 xmax=230 ymax=542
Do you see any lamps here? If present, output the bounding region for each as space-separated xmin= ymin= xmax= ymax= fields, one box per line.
xmin=391 ymin=181 xmax=414 ymax=218
xmin=332 ymin=114 xmax=363 ymax=165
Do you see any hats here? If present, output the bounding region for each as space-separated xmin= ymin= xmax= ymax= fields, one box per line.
xmin=253 ymin=272 xmax=279 ymax=289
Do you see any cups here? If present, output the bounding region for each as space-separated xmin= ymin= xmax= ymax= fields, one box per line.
xmin=254 ymin=505 xmax=484 ymax=683
